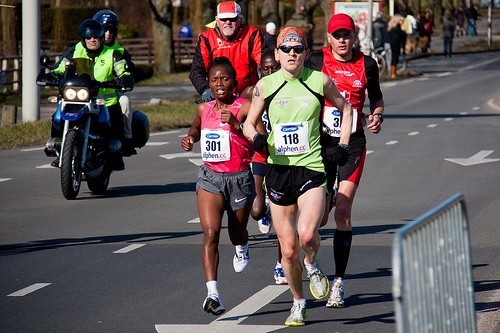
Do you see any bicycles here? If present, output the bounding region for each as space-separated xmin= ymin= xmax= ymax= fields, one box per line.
xmin=361 ymin=35 xmax=386 ymax=78
xmin=378 ymin=42 xmax=407 ymax=74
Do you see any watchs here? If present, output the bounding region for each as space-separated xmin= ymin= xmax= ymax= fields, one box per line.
xmin=374 ymin=113 xmax=383 ymax=124
xmin=238 ymin=123 xmax=244 ymax=132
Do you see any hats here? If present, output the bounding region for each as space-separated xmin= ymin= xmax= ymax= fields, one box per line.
xmin=216 ymin=1 xmax=241 ymax=19
xmin=327 ymin=14 xmax=354 ymax=34
xmin=276 ymin=26 xmax=307 ymax=49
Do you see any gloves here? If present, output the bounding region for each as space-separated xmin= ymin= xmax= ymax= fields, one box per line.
xmin=35 ymin=71 xmax=51 ymax=87
xmin=202 ymin=88 xmax=215 ymax=103
xmin=118 ymin=75 xmax=134 ymax=89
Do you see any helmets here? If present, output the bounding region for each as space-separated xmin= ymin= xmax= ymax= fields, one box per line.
xmin=92 ymin=10 xmax=119 ymax=33
xmin=77 ymin=20 xmax=105 ymax=46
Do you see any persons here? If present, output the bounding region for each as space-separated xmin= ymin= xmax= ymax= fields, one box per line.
xmin=465 ymin=5 xmax=478 ymax=37
xmin=264 ymin=22 xmax=282 ymax=72
xmin=242 ymin=27 xmax=353 ymax=326
xmin=92 ymin=10 xmax=136 ymax=156
xmin=182 ymin=57 xmax=269 ymax=316
xmin=456 ymin=7 xmax=464 ymax=37
xmin=387 ymin=14 xmax=404 ymax=79
xmin=442 ymin=16 xmax=455 ymax=57
xmin=370 ymin=12 xmax=386 ymax=65
xmin=36 ymin=20 xmax=132 ymax=171
xmin=188 ymin=1 xmax=265 ymax=104
xmin=305 ymin=12 xmax=384 ymax=308
xmin=240 ymin=49 xmax=282 ymax=235
xmin=403 ymin=10 xmax=435 ymax=53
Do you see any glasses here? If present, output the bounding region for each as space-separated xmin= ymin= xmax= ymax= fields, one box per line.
xmin=84 ymin=36 xmax=100 ymax=40
xmin=219 ymin=17 xmax=239 ymax=22
xmin=102 ymin=25 xmax=115 ymax=34
xmin=276 ymin=45 xmax=306 ymax=55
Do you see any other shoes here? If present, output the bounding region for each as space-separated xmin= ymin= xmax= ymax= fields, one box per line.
xmin=43 ymin=142 xmax=61 ymax=156
xmin=122 ymin=138 xmax=133 ymax=156
xmin=108 ymin=139 xmax=122 ymax=151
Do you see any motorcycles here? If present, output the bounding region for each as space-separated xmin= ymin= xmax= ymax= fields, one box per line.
xmin=35 ymin=55 xmax=150 ymax=201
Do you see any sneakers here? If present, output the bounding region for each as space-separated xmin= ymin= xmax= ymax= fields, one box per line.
xmin=232 ymin=228 xmax=250 ymax=273
xmin=284 ymin=302 xmax=309 ymax=325
xmin=331 ymin=187 xmax=339 ymax=208
xmin=303 ymin=255 xmax=329 ymax=299
xmin=325 ymin=281 xmax=345 ymax=307
xmin=274 ymin=267 xmax=289 ymax=284
xmin=203 ymin=293 xmax=226 ymax=316
xmin=256 ymin=194 xmax=272 ymax=234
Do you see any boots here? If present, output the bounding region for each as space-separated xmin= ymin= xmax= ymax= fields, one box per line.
xmin=389 ymin=66 xmax=397 ymax=80
